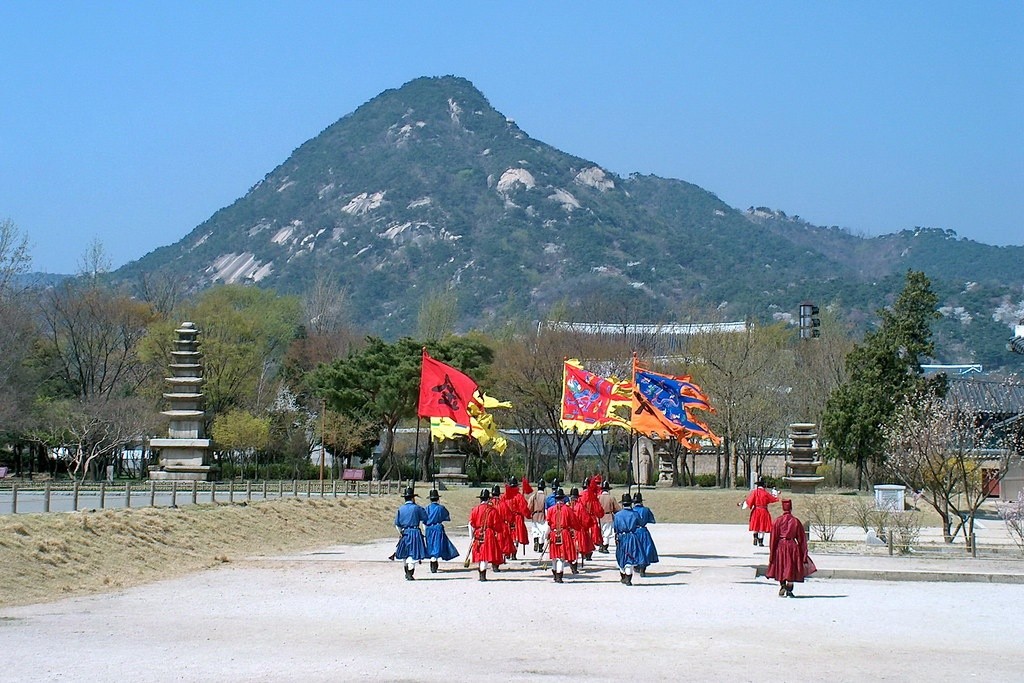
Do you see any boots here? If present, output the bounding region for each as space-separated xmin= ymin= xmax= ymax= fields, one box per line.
xmin=753 ymin=533 xmax=757 ymax=546
xmin=551 ymin=544 xmax=609 ymax=582
xmin=478 ymin=549 xmax=517 ymax=582
xmin=786 ymin=583 xmax=796 ymax=598
xmin=758 ymin=538 xmax=764 ymax=546
xmin=619 ymin=571 xmax=632 ymax=586
xmin=534 ymin=537 xmax=543 ymax=552
xmin=430 ymin=561 xmax=438 ymax=573
xmin=404 ymin=566 xmax=415 ymax=580
xmin=779 ymin=581 xmax=786 ymax=596
xmin=634 ymin=566 xmax=646 ymax=577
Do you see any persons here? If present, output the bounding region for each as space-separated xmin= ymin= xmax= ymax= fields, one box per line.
xmin=397 ymin=476 xmax=659 ymax=586
xmin=740 ymin=476 xmax=779 ymax=547
xmin=765 ymin=497 xmax=817 ymax=597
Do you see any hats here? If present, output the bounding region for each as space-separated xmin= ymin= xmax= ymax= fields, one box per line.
xmin=550 ymin=478 xmax=563 ymax=488
xmin=617 ymin=493 xmax=636 ymax=503
xmin=400 ymin=488 xmax=418 ymax=497
xmin=551 ymin=487 xmax=566 ymax=498
xmin=632 ymin=492 xmax=645 ymax=501
xmin=754 ymin=477 xmax=764 ymax=484
xmin=782 ymin=499 xmax=792 ymax=511
xmin=476 ymin=489 xmax=493 ymax=498
xmin=582 ymin=477 xmax=590 ymax=488
xmin=600 ymin=480 xmax=613 ymax=490
xmin=427 ymin=490 xmax=441 ymax=498
xmin=489 ymin=485 xmax=504 ymax=494
xmin=508 ymin=476 xmax=520 ymax=485
xmin=569 ymin=486 xmax=582 ymax=497
xmin=538 ymin=479 xmax=547 ymax=488
